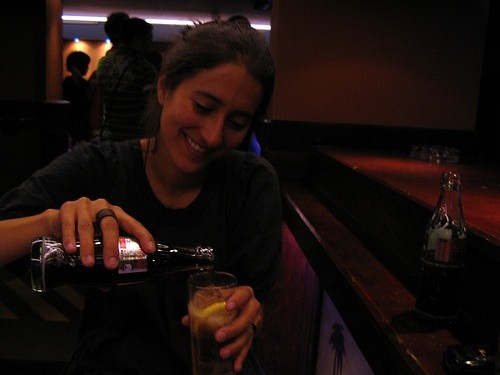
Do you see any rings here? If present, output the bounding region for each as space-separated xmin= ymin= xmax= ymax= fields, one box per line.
xmin=96 ymin=208 xmax=118 ymax=226
xmin=250 ymin=322 xmax=258 ymax=337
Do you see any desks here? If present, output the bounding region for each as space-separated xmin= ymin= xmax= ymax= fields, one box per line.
xmin=326 ymin=150 xmax=500 ymax=249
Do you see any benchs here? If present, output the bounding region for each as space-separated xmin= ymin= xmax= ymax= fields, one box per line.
xmin=260 ymin=117 xmax=478 ymax=184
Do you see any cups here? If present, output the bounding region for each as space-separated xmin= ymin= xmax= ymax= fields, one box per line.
xmin=188 ymin=271 xmax=238 ymax=375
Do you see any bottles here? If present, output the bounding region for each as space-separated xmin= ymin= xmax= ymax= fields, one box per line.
xmin=29 ymin=237 xmax=215 ymax=292
xmin=421 ymin=170 xmax=468 ymax=320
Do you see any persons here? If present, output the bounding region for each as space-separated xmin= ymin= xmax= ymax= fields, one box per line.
xmin=88 ymin=16 xmax=164 ymax=144
xmin=0 ymin=13 xmax=285 ymax=375
xmin=58 ymin=50 xmax=96 ymax=150
